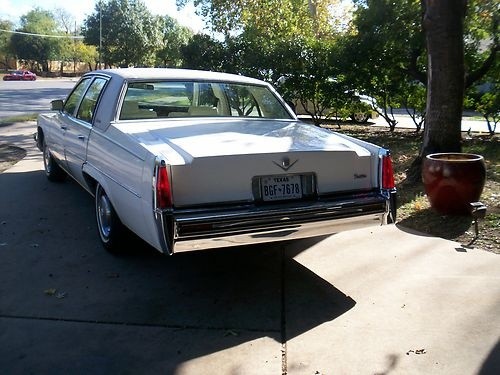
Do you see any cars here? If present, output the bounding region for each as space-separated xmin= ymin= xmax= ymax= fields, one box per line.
xmin=33 ymin=66 xmax=399 ymax=261
xmin=3 ymin=67 xmax=37 ymax=80
xmin=271 ymin=69 xmax=383 ymax=124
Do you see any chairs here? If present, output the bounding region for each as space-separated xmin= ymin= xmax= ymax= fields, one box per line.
xmin=119 ymin=100 xmax=219 ymax=120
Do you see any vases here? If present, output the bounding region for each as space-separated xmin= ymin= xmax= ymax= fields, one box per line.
xmin=421 ymin=152 xmax=487 ymax=216
